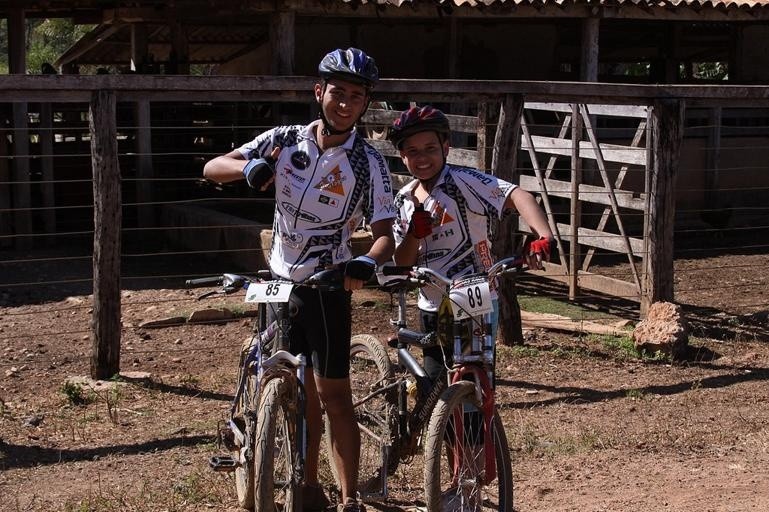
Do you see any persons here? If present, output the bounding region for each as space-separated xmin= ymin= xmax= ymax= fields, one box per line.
xmin=203 ymin=43 xmax=397 ymax=511
xmin=382 ymin=103 xmax=559 ymax=512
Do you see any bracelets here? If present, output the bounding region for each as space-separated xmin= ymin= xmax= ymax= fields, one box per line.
xmin=399 ymin=238 xmax=420 ymax=258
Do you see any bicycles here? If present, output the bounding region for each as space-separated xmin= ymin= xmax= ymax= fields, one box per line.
xmin=345 ymin=240 xmax=561 ymax=510
xmin=181 ymin=256 xmax=384 ymax=512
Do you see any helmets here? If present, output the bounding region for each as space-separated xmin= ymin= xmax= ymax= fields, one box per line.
xmin=390 ymin=106 xmax=450 ymax=150
xmin=319 ymin=47 xmax=378 ymax=89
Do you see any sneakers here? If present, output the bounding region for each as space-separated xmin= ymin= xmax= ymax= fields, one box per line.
xmin=274 ymin=482 xmax=330 ymax=512
xmin=337 ymin=497 xmax=366 ymax=512
xmin=442 ymin=488 xmax=474 ymax=512
